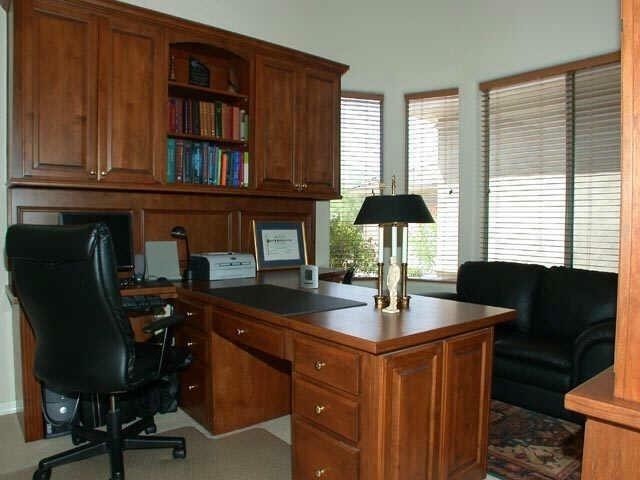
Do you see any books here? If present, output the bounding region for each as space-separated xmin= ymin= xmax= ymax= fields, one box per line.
xmin=167 ymin=96 xmax=249 ymax=189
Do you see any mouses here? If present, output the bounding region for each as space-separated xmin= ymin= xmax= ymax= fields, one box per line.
xmin=157 ymin=277 xmax=170 ymax=285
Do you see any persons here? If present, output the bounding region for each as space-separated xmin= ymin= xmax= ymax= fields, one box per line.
xmin=386 ymin=257 xmax=401 ymax=310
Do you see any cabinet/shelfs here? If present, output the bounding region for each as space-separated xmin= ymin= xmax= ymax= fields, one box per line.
xmin=254 ymin=53 xmax=341 ymax=194
xmin=6 ymin=0 xmax=162 ymax=183
xmin=168 ymin=81 xmax=248 ymax=145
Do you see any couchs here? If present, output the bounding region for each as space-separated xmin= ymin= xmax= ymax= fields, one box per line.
xmin=415 ymin=261 xmax=618 ymax=426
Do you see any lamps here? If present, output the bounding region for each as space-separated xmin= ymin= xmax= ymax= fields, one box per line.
xmin=353 ymin=175 xmax=436 ymax=310
xmin=170 ymin=227 xmax=197 ymax=292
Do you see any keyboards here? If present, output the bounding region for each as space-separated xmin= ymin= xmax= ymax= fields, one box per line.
xmin=119 ymin=295 xmax=161 ymax=309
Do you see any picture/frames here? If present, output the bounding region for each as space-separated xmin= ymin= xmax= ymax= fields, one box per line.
xmin=252 ymin=219 xmax=309 ymax=272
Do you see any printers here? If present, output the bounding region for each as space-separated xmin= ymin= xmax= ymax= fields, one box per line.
xmin=189 ymin=252 xmax=256 ymax=280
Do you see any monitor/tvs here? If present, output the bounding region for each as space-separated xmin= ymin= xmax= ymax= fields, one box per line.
xmin=60 ymin=211 xmax=135 ymax=271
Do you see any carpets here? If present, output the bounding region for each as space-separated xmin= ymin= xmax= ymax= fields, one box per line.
xmin=486 ymin=398 xmax=582 ymax=479
xmin=13 ymin=425 xmax=291 ymax=480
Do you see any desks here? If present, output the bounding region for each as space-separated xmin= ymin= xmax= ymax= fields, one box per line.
xmin=172 ymin=272 xmax=518 ymax=480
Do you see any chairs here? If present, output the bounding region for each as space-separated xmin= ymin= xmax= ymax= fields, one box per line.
xmin=6 ymin=222 xmax=187 ymax=480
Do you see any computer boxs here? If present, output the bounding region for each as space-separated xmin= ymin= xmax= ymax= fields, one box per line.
xmin=42 ymin=384 xmax=83 ymax=439
